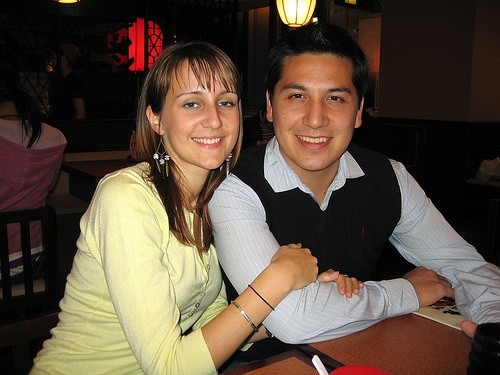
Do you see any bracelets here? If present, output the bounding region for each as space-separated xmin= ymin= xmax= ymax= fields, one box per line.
xmin=230 ymin=301 xmax=259 ymax=331
xmin=248 ymin=285 xmax=274 ymax=311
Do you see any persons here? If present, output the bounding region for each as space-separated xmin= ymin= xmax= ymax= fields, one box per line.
xmin=0 ymin=64 xmax=67 ymax=285
xmin=26 ymin=41 xmax=366 ymax=375
xmin=210 ymin=21 xmax=500 ymax=374
xmin=39 ymin=45 xmax=87 ymax=122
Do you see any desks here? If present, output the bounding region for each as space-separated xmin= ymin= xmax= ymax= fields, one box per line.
xmin=61 ymin=150 xmax=141 ymax=185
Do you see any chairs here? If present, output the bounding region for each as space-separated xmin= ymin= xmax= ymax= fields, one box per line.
xmin=0 ymin=314 xmax=59 ymax=375
xmin=0 ymin=207 xmax=58 ymax=321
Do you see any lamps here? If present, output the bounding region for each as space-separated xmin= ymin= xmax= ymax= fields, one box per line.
xmin=276 ymin=0 xmax=317 ymax=28
